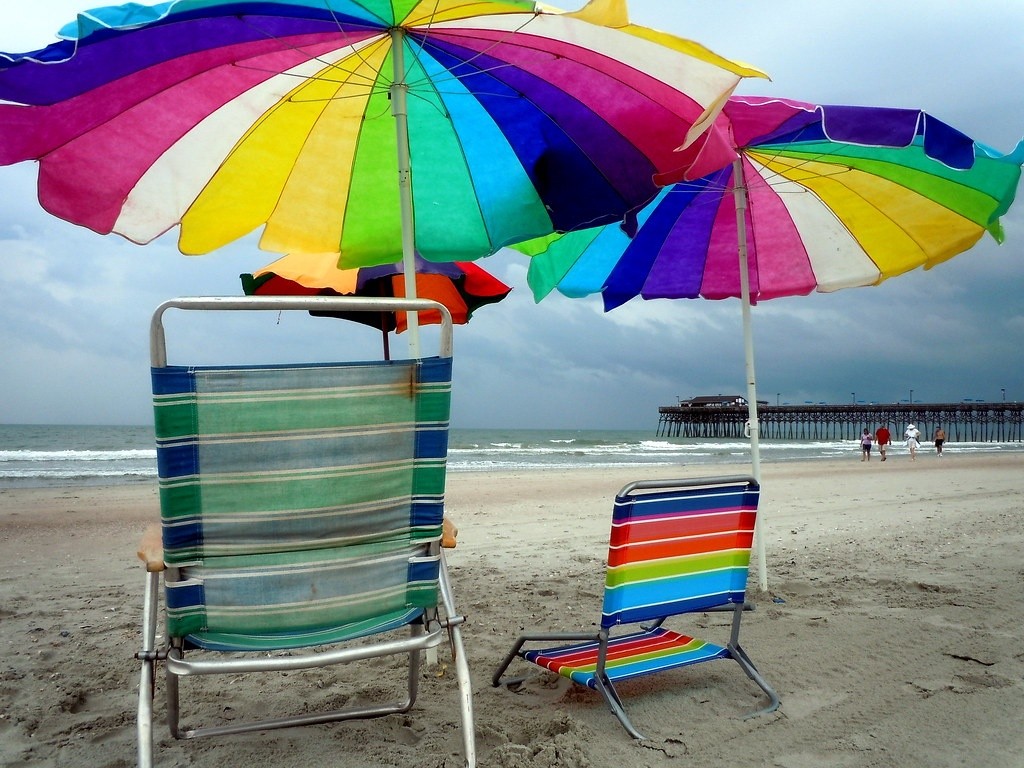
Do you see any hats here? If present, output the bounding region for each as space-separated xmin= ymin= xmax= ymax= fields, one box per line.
xmin=907 ymin=423 xmax=915 ymax=429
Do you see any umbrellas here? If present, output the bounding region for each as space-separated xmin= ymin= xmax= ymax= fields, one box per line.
xmin=514 ymin=95 xmax=1023 ymax=589
xmin=239 ymin=250 xmax=514 ymax=360
xmin=0 ymin=0 xmax=771 ymax=666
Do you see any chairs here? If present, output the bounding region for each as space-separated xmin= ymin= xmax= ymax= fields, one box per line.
xmin=137 ymin=296 xmax=481 ymax=768
xmin=491 ymin=475 xmax=782 ymax=738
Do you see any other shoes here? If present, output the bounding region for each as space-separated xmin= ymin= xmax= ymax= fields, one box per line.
xmin=911 ymin=455 xmax=916 ymax=461
xmin=881 ymin=458 xmax=886 ymax=461
xmin=937 ymin=453 xmax=943 ymax=457
xmin=861 ymin=459 xmax=865 ymax=462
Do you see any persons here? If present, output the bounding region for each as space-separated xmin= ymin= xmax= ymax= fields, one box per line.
xmin=904 ymin=424 xmax=921 ymax=460
xmin=860 ymin=428 xmax=873 ymax=462
xmin=932 ymin=426 xmax=945 ymax=457
xmin=875 ymin=424 xmax=891 ymax=461
